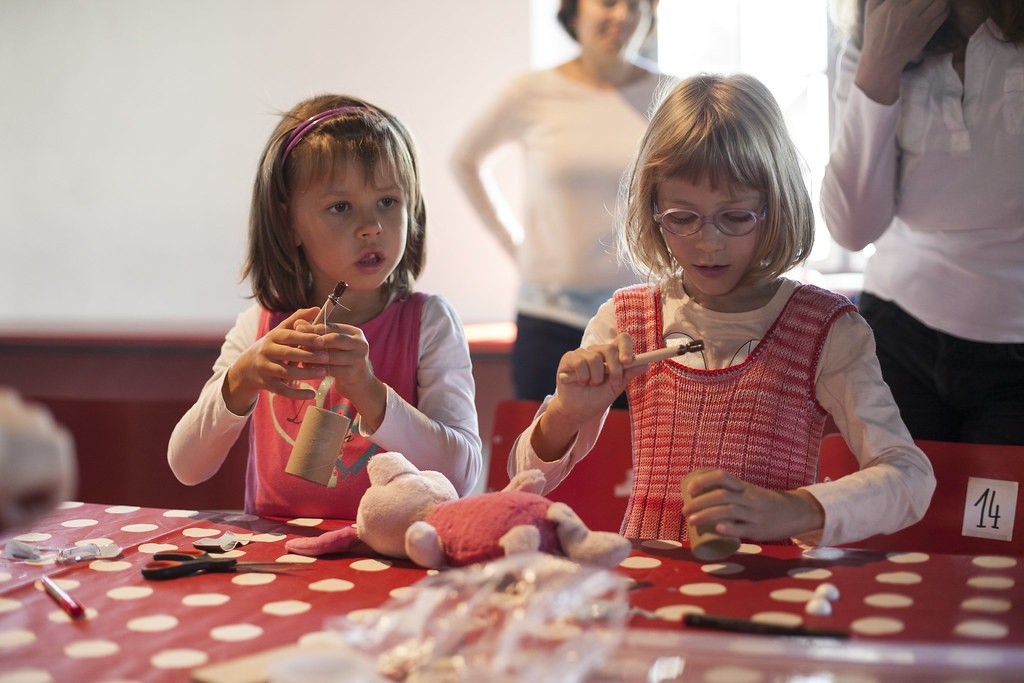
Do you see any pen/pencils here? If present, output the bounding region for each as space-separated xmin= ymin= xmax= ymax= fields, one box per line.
xmin=557 ymin=339 xmax=706 ymax=385
xmin=275 ymin=281 xmax=348 ymax=384
xmin=681 ymin=609 xmax=850 ymax=640
xmin=40 ymin=574 xmax=84 ymax=619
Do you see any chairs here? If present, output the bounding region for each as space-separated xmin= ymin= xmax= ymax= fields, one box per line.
xmin=481 ymin=402 xmax=635 ymax=536
xmin=812 ymin=429 xmax=1023 ymax=555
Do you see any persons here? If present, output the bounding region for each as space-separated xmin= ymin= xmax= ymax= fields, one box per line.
xmin=819 ymin=0 xmax=1024 ymax=457
xmin=501 ymin=69 xmax=939 ymax=554
xmin=170 ymin=92 xmax=484 ymax=524
xmin=450 ymin=2 xmax=682 ymax=413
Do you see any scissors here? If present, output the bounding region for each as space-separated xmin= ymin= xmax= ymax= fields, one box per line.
xmin=140 ymin=550 xmax=317 ymax=582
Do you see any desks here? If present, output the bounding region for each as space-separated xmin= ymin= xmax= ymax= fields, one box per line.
xmin=2 ymin=491 xmax=1024 ymax=683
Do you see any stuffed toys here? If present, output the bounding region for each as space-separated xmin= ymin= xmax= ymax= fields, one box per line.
xmin=283 ymin=451 xmax=633 ymax=573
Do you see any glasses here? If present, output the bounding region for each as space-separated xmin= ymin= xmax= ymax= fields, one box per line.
xmin=654 ymin=202 xmax=770 ymax=238
